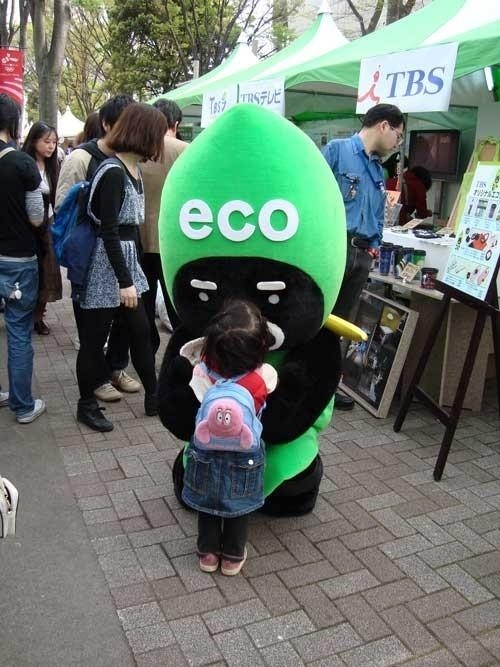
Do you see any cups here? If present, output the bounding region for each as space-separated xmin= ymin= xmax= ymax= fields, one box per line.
xmin=489 ymin=204 xmax=497 ymax=217
xmin=420 ymin=267 xmax=439 ymax=288
xmin=376 ymin=241 xmax=426 ymax=282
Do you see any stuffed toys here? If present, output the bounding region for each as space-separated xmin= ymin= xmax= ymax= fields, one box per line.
xmin=159 ymin=102 xmax=365 ymax=519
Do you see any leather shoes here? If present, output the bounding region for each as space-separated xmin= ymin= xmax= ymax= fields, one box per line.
xmin=34 ymin=319 xmax=49 ymax=335
xmin=335 ymin=394 xmax=354 ymax=410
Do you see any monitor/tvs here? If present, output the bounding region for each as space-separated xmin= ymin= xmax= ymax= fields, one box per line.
xmin=409 ymin=130 xmax=462 ymax=183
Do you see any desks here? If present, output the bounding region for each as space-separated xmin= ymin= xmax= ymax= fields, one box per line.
xmin=368 ymin=225 xmax=490 ymax=416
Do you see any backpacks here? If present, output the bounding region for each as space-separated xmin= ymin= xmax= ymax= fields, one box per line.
xmin=194 ymin=382 xmax=263 ymax=454
xmin=51 ymin=182 xmax=89 ymax=267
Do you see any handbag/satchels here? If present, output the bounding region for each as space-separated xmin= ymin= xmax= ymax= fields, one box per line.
xmin=37 ymin=228 xmax=63 ymax=302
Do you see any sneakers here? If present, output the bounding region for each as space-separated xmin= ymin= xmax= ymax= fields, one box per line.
xmin=198 ymin=544 xmax=248 ymax=576
xmin=78 ymin=398 xmax=113 ymax=432
xmin=16 ymin=399 xmax=45 ymax=423
xmin=108 ymin=370 xmax=141 ymax=392
xmin=0 ymin=392 xmax=10 ymax=407
xmin=95 ymin=382 xmax=123 ymax=401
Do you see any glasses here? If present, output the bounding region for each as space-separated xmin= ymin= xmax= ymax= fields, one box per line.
xmin=392 ymin=125 xmax=404 ymax=145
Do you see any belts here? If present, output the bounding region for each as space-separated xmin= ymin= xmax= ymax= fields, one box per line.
xmin=347 ymin=234 xmax=366 ymax=248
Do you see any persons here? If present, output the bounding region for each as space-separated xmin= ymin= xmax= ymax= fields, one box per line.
xmin=381 ymin=150 xmax=432 ymax=226
xmin=321 ymin=104 xmax=406 ymax=409
xmin=1 ymin=88 xmax=191 ymax=428
xmin=180 ymin=297 xmax=278 ymax=574
